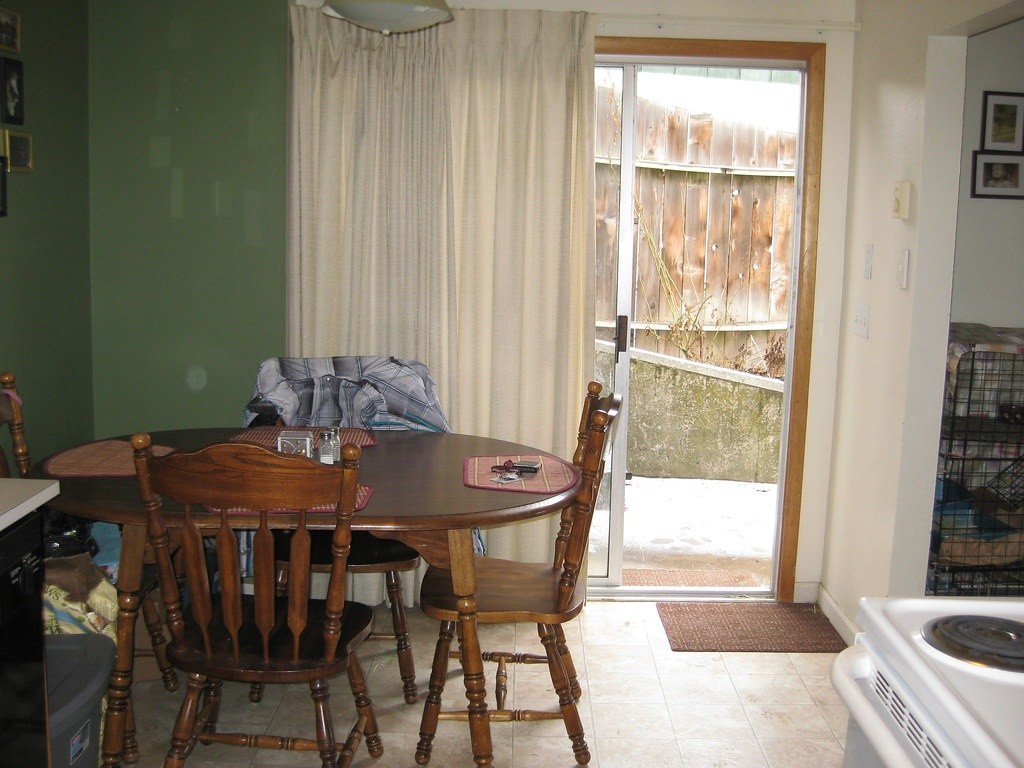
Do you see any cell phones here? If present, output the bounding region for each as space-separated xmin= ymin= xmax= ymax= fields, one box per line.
xmin=512 ymin=460 xmax=542 ymax=473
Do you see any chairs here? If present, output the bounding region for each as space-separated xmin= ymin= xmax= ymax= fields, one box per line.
xmin=251 ymin=353 xmax=421 ymax=706
xmin=127 ymin=428 xmax=383 ymax=767
xmin=0 ymin=370 xmax=179 ymax=693
xmin=415 ymin=382 xmax=626 ymax=764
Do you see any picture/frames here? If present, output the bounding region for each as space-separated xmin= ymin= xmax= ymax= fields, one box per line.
xmin=979 ymin=89 xmax=1023 ymax=153
xmin=970 ymin=149 xmax=1023 ymax=199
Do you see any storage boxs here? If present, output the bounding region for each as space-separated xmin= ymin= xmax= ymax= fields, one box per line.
xmin=45 ymin=633 xmax=117 ymax=768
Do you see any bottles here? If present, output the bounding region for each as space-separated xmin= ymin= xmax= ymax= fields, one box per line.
xmin=329 ymin=427 xmax=341 ymax=460
xmin=318 ymin=432 xmax=333 ymax=464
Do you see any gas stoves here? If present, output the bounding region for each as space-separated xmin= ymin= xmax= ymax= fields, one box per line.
xmin=854 ymin=595 xmax=1024 ymax=768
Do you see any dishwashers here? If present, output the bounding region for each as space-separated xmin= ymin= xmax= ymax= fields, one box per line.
xmin=841 ymin=632 xmax=974 ymax=768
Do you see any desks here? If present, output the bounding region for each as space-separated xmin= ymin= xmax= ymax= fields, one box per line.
xmin=35 ymin=428 xmax=582 ymax=765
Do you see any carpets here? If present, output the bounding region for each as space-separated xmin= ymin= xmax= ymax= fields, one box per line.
xmin=617 ymin=565 xmax=760 ymax=588
xmin=657 ymin=599 xmax=847 ymax=655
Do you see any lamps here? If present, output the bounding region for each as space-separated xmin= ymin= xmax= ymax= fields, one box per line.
xmin=322 ymin=0 xmax=452 ymax=35
